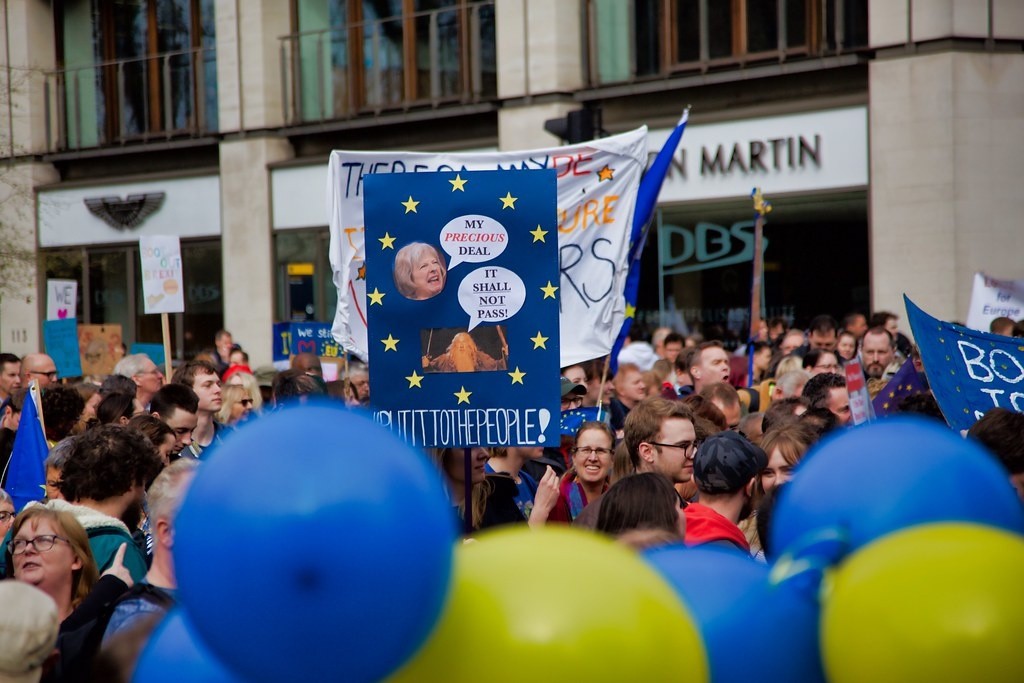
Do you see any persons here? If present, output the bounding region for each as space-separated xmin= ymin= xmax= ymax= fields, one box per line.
xmin=426 ymin=311 xmax=1024 ymax=558
xmin=395 ymin=241 xmax=446 ymax=302
xmin=0 ymin=332 xmax=369 ymax=683
xmin=422 ymin=332 xmax=510 ymax=371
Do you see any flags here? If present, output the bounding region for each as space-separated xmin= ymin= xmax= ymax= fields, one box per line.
xmin=603 ymin=108 xmax=692 ymax=374
xmin=4 ymin=384 xmax=51 ymax=514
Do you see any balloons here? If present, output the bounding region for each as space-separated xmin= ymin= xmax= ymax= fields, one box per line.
xmin=133 ymin=606 xmax=239 ymax=683
xmin=639 ymin=540 xmax=825 ymax=683
xmin=772 ymin=408 xmax=1024 ymax=579
xmin=173 ymin=396 xmax=460 ymax=683
xmin=386 ymin=521 xmax=710 ymax=683
xmin=817 ymin=523 xmax=1024 ymax=683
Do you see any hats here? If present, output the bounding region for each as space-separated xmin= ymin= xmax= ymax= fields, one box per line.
xmin=618 ymin=342 xmax=660 ymax=371
xmin=561 ymin=376 xmax=587 ymax=397
xmin=692 ymin=430 xmax=768 ymax=491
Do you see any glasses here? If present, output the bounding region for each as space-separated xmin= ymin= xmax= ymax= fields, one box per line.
xmin=637 ymin=441 xmax=697 ymax=457
xmin=30 ymin=370 xmax=56 ymax=380
xmin=234 ymin=399 xmax=253 ymax=407
xmin=814 ymin=364 xmax=837 ymax=372
xmin=0 ymin=511 xmax=16 ymax=525
xmin=576 ymin=447 xmax=614 ymax=455
xmin=561 ymin=397 xmax=583 ymax=411
xmin=5 ymin=535 xmax=70 ymax=555
